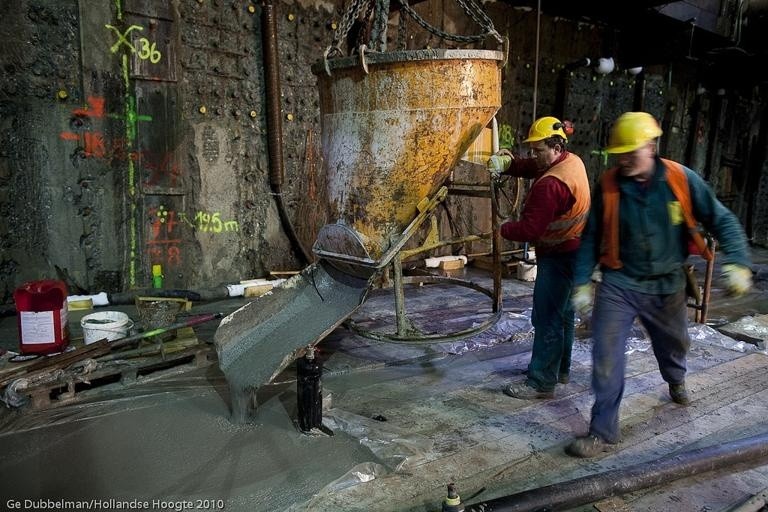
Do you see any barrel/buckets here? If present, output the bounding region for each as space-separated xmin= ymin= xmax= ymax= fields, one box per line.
xmin=81 ymin=310 xmax=133 ymax=346
xmin=13 ymin=280 xmax=68 ymax=353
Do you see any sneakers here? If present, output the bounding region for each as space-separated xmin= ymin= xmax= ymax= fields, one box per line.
xmin=668 ymin=382 xmax=689 ymax=406
xmin=568 ymin=432 xmax=620 ymax=458
xmin=505 ymin=363 xmax=570 ymax=400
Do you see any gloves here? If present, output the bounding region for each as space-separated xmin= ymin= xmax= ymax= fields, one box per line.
xmin=720 ymin=262 xmax=753 ymax=300
xmin=486 ymin=154 xmax=512 ymax=180
xmin=573 ymin=283 xmax=595 ymax=320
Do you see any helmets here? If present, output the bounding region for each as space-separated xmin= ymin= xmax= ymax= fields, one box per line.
xmin=522 ymin=116 xmax=569 ymax=145
xmin=606 ymin=111 xmax=663 ymax=155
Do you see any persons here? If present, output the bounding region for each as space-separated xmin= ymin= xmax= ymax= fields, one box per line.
xmin=488 ymin=115 xmax=592 ymax=399
xmin=565 ymin=110 xmax=752 ymax=455
